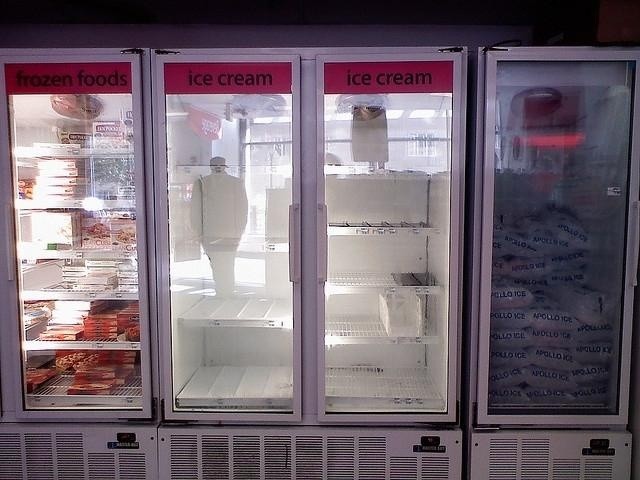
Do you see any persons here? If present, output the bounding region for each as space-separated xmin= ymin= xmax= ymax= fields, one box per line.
xmin=190 ymin=157 xmax=248 ymax=298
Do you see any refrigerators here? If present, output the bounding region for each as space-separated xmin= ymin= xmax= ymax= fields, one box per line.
xmin=0 ymin=44 xmax=638 ymax=480
